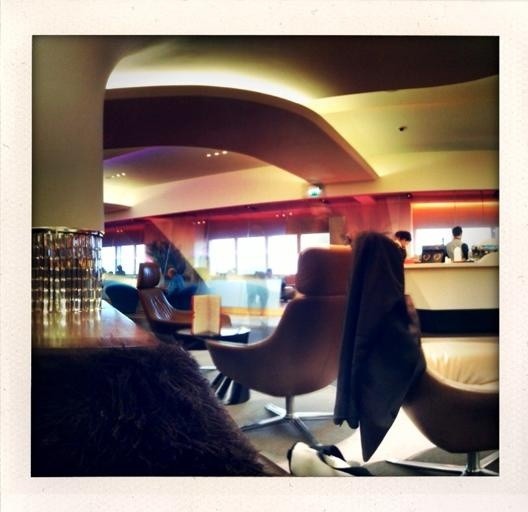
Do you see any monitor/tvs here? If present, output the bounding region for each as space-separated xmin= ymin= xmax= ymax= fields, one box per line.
xmin=422 ymin=246 xmax=449 ymax=262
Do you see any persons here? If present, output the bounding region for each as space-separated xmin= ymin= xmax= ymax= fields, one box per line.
xmin=115 ymin=265 xmax=125 ymax=275
xmin=158 ymin=265 xmax=187 ymax=299
xmin=262 ymin=268 xmax=287 ymax=292
xmin=446 ymin=225 xmax=472 ymax=259
xmin=391 ymin=231 xmax=410 ymax=251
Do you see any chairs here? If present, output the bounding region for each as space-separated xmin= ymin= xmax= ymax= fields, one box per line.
xmin=333 ymin=232 xmax=500 ymax=477
xmin=105 ymin=231 xmax=297 ymax=406
xmin=205 ymin=244 xmax=358 ymax=448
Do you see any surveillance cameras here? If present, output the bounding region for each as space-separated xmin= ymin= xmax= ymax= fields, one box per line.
xmin=400 ymin=127 xmax=404 ymax=131
